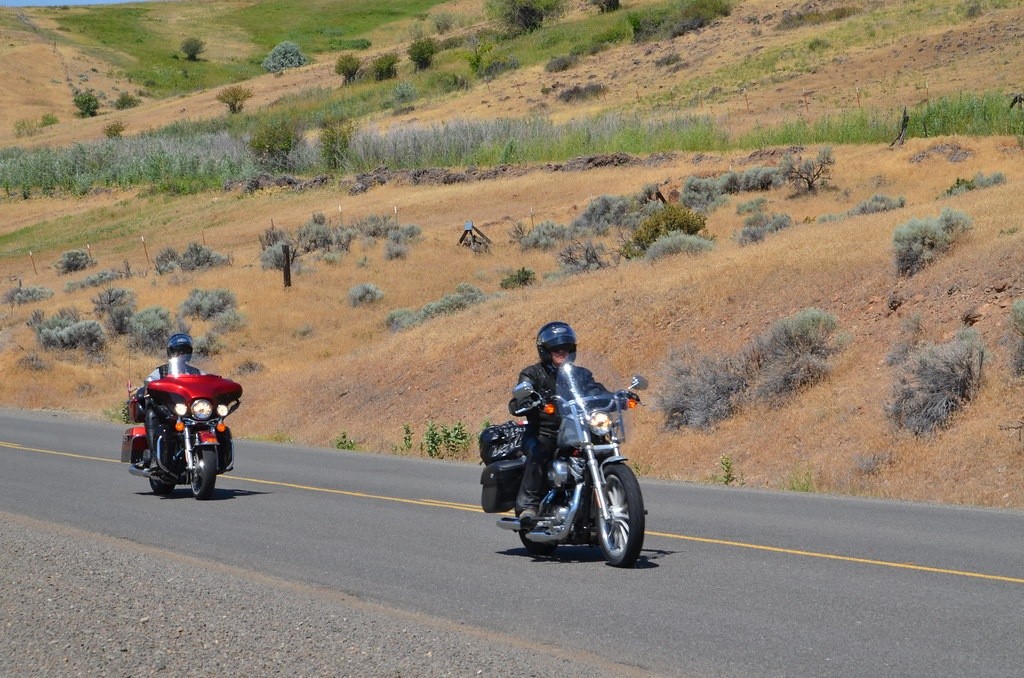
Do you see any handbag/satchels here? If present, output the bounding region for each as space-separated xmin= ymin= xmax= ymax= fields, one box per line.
xmin=480 ymin=421 xmax=528 ymax=467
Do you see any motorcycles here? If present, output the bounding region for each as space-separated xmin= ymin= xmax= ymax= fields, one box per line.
xmin=481 ymin=349 xmax=648 ymax=568
xmin=120 ymin=353 xmax=243 ymax=500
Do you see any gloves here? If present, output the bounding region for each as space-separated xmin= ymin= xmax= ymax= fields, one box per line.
xmin=616 ymin=391 xmax=640 ymax=410
xmin=515 ymin=396 xmax=537 ymax=412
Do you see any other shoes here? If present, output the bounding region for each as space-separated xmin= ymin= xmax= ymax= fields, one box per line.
xmin=518 ymin=508 xmax=536 ymax=522
xmin=150 ymin=451 xmax=158 ymax=471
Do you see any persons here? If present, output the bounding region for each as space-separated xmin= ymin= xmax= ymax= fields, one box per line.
xmin=145 ymin=333 xmax=232 ymax=474
xmin=509 ymin=320 xmax=640 ymax=526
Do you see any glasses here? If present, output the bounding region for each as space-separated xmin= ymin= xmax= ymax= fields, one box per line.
xmin=171 ymin=346 xmax=191 ymax=354
xmin=549 ymin=343 xmax=574 ymax=351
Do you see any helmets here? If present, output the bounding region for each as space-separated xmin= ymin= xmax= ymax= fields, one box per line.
xmin=537 ymin=321 xmax=577 ymax=372
xmin=166 ymin=334 xmax=193 ymax=362
